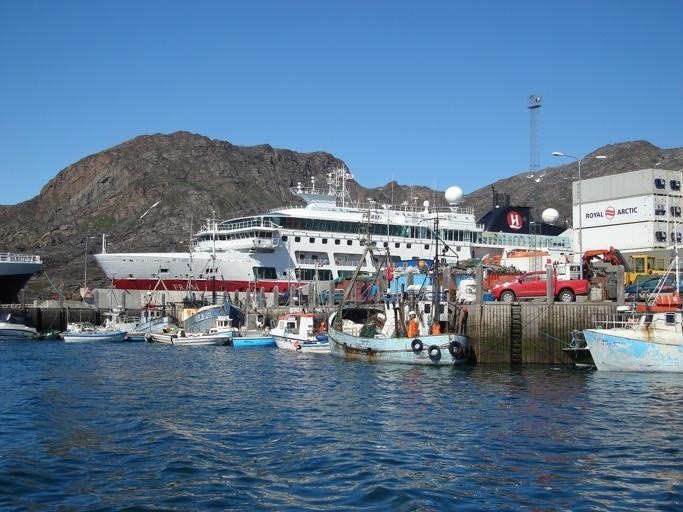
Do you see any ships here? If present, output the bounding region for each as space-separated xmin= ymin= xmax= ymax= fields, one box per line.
xmin=93 ymin=164 xmax=573 ymax=311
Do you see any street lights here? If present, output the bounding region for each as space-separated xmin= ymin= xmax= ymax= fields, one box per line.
xmin=552 ymin=152 xmax=608 ymax=279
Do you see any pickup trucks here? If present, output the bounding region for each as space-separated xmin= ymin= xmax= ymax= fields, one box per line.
xmin=491 ymin=271 xmax=590 ymax=303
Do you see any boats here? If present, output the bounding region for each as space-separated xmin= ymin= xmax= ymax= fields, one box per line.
xmin=37 ymin=329 xmax=61 ymax=341
xmin=229 ymin=335 xmax=276 ymax=348
xmin=0 ymin=312 xmax=38 ymax=339
xmin=267 ymin=309 xmax=333 ymax=353
xmin=63 ymin=295 xmax=235 ymax=349
xmin=562 ymin=312 xmax=683 ymax=373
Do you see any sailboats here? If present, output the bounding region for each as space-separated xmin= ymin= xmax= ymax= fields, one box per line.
xmin=324 ymin=191 xmax=473 ymax=367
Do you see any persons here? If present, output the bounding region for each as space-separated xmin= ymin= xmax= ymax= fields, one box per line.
xmin=361 ymin=281 xmax=377 ymax=304
xmin=431 ymin=318 xmax=441 ymax=335
xmin=407 ymin=311 xmax=419 ymax=337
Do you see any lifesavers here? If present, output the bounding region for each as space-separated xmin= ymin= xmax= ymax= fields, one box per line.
xmin=316 ymin=335 xmax=328 ymax=341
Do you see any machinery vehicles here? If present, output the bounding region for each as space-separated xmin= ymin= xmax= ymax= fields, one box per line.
xmin=582 ymin=247 xmax=683 ymax=288
